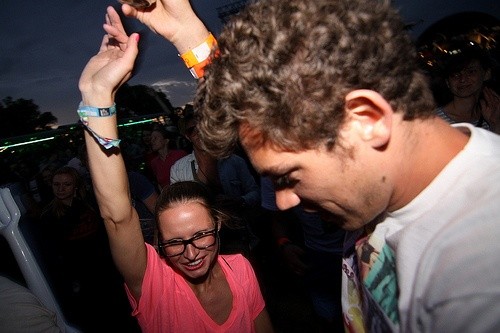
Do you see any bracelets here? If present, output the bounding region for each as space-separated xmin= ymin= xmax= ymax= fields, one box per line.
xmin=78 ymin=101 xmax=121 ymax=149
xmin=181 ymin=35 xmax=220 ymax=79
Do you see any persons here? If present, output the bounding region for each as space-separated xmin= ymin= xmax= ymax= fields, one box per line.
xmin=78 ymin=5 xmax=273 ymax=333
xmin=122 ymin=112 xmax=345 ymax=333
xmin=437 ymin=40 xmax=500 ymax=132
xmin=118 ymin=1 xmax=500 ymax=333
xmin=0 ymin=140 xmax=111 ymax=273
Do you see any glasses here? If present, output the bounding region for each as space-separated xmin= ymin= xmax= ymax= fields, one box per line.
xmin=158 ymin=222 xmax=218 ymax=257
